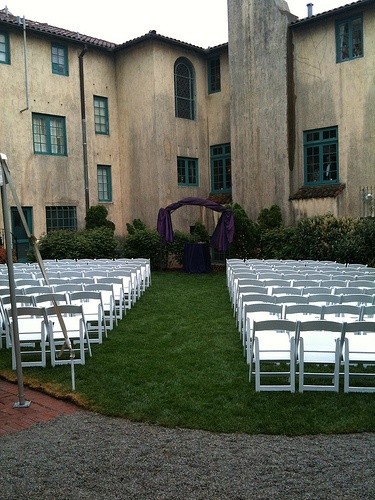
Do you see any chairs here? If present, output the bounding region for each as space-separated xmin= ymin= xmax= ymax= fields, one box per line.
xmin=0 ymin=257 xmax=152 ymax=370
xmin=225 ymin=258 xmax=375 ymax=394
